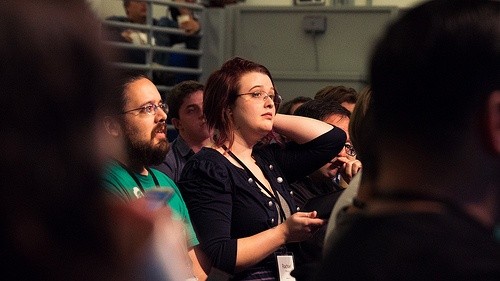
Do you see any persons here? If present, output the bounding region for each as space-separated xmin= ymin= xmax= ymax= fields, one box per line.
xmin=102 ymin=75 xmax=211 ymax=281
xmin=180 ymin=57 xmax=347 ymax=281
xmin=103 ymin=0 xmax=375 ymax=248
xmin=0 ymin=0 xmax=171 ymax=281
xmin=323 ymin=0 xmax=500 ymax=281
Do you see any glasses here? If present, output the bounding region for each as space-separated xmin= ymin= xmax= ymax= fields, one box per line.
xmin=344 ymin=144 xmax=357 ymax=156
xmin=125 ymin=103 xmax=169 ymax=115
xmin=238 ymin=90 xmax=282 ymax=104
